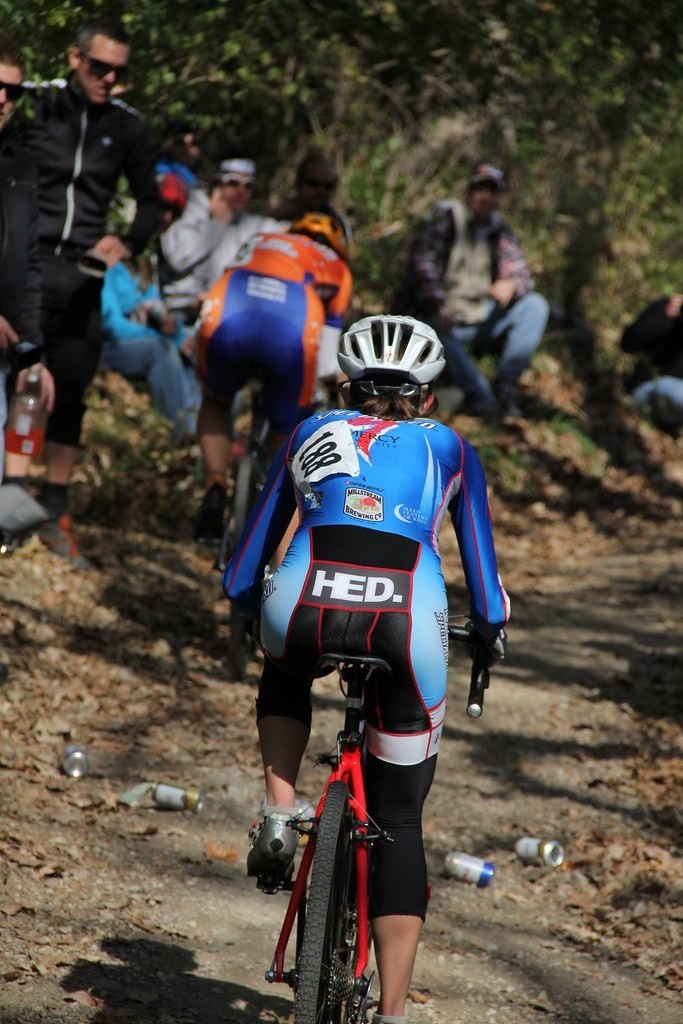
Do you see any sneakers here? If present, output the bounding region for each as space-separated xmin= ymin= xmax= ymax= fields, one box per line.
xmin=37 ymin=511 xmax=89 ymax=571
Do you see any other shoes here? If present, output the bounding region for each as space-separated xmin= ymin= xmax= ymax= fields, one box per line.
xmin=246 ymin=799 xmax=300 ymax=879
xmin=194 ymin=483 xmax=229 ymax=545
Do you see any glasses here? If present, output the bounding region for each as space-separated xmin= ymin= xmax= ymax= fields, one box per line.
xmin=305 ymin=177 xmax=336 ymax=191
xmin=158 ymin=198 xmax=183 ymax=219
xmin=79 ymin=52 xmax=132 ymax=87
xmin=474 ymin=184 xmax=503 ymax=195
xmin=0 ymin=82 xmax=28 ymax=104
xmin=211 ymin=175 xmax=256 ymax=192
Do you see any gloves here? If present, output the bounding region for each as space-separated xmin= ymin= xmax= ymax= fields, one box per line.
xmin=467 ymin=629 xmax=508 ymax=663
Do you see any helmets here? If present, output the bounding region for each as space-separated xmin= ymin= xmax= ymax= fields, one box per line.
xmin=290 ymin=211 xmax=350 ymax=258
xmin=336 ymin=313 xmax=446 ymax=390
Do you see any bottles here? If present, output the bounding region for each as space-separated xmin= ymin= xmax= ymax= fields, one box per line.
xmin=7 ymin=365 xmax=42 ymax=438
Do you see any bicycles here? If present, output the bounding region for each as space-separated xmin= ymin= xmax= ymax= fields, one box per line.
xmin=254 ymin=577 xmax=494 ymax=1024
xmin=201 ymin=348 xmax=322 ymax=676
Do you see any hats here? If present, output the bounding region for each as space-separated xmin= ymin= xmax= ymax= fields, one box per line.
xmin=155 ymin=169 xmax=192 ymax=211
xmin=465 ymin=164 xmax=507 ymax=185
xmin=295 ymin=147 xmax=343 ymax=181
xmin=214 ymin=158 xmax=257 ymax=181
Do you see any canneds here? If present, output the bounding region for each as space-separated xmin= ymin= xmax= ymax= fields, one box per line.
xmin=514 ymin=837 xmax=564 ymax=867
xmin=443 ymin=851 xmax=495 ymax=887
xmin=61 ymin=744 xmax=87 ymax=778
xmin=151 ymin=781 xmax=206 ymax=816
xmin=259 ymin=797 xmax=315 ymax=825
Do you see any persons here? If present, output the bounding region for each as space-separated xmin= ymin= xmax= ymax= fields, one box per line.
xmin=102 ymin=260 xmax=199 ymax=436
xmin=621 ymin=294 xmax=683 ymax=428
xmin=187 ymin=210 xmax=353 ymax=571
xmin=272 ymin=153 xmax=340 ymax=218
xmin=414 ymin=163 xmax=551 ymax=423
xmin=220 ymin=315 xmax=512 ymax=1024
xmin=0 ymin=17 xmax=163 ymax=568
xmin=158 ymin=156 xmax=281 ymax=310
